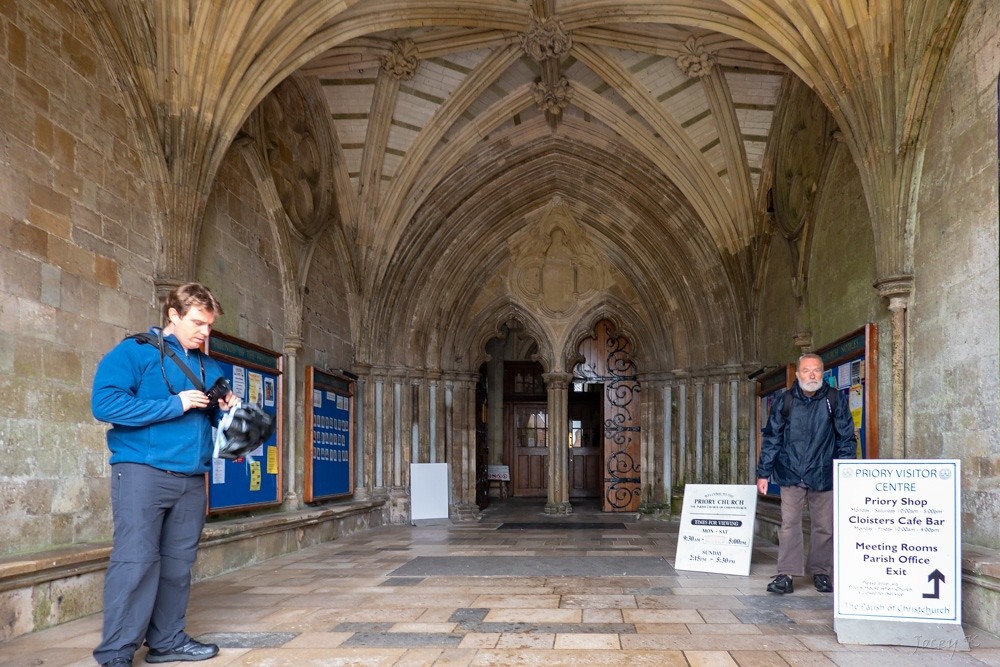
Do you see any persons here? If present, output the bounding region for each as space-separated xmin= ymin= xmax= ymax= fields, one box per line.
xmin=757 ymin=353 xmax=858 ymax=594
xmin=90 ymin=283 xmax=241 ymax=667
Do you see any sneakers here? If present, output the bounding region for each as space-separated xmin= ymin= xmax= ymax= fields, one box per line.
xmin=766 ymin=573 xmax=794 ymax=594
xmin=101 ymin=657 xmax=135 ymax=667
xmin=812 ymin=575 xmax=834 ymax=593
xmin=143 ymin=638 xmax=219 ymax=663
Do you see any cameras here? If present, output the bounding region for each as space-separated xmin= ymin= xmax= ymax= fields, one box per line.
xmin=196 ymin=376 xmax=232 ymax=413
